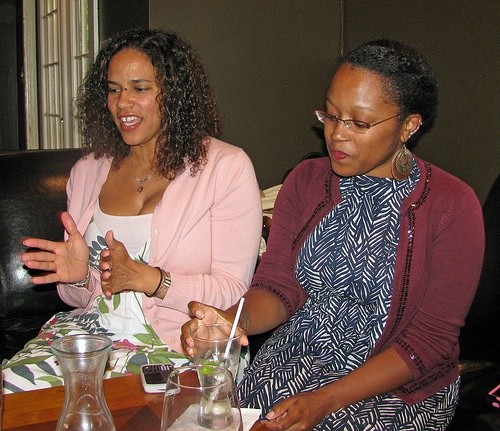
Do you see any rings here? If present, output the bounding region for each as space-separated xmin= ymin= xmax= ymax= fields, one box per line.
xmin=108 ymin=263 xmax=112 ymax=271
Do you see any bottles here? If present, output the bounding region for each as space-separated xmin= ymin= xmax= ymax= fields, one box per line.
xmin=50 ymin=333 xmax=116 ymax=431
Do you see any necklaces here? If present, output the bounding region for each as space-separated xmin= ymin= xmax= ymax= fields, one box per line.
xmin=130 ymin=160 xmax=148 ymax=192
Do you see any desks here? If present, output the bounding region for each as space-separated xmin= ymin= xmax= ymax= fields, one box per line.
xmin=2 ymin=370 xmax=265 ymax=430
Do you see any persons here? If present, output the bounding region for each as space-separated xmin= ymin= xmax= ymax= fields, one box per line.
xmin=181 ymin=40 xmax=485 ymax=431
xmin=0 ymin=29 xmax=264 ymax=390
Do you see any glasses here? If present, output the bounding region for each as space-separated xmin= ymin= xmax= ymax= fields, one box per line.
xmin=316 ymin=109 xmax=399 ymax=134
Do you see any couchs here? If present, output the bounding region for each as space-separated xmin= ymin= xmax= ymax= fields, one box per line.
xmin=0 ymin=146 xmax=92 ymax=358
xmin=437 ymin=176 xmax=500 ymax=431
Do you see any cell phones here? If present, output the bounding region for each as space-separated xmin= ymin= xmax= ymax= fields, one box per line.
xmin=140 ymin=363 xmax=181 ymax=394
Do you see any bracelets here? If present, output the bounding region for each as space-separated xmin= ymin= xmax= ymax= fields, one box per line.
xmin=72 ymin=269 xmax=90 ymax=287
xmin=144 ymin=266 xmax=171 ymax=298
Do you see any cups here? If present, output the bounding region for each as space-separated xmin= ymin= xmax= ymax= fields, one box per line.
xmin=161 ymin=365 xmax=243 ymax=431
xmin=191 ymin=324 xmax=242 ymax=429
